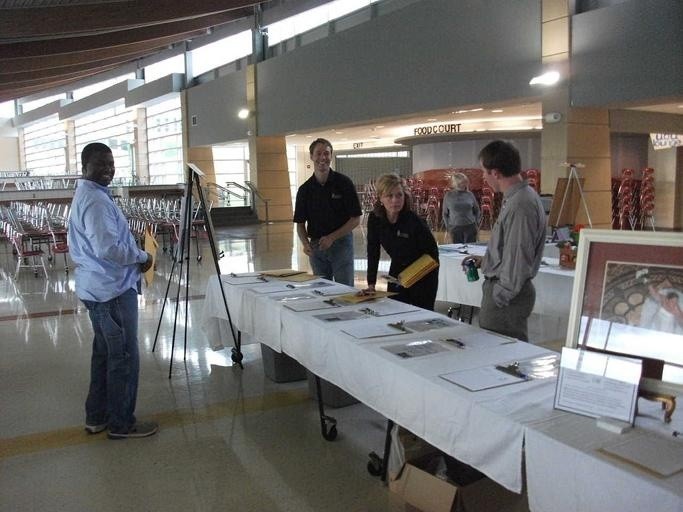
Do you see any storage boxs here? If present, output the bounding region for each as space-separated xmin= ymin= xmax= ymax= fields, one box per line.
xmin=394 ymin=460 xmax=501 ymax=511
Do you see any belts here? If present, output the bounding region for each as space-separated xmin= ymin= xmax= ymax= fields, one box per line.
xmin=484 ymin=275 xmax=500 ymax=281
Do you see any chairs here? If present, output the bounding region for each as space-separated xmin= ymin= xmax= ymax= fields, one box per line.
xmin=357 ymin=169 xmax=540 ymax=227
xmin=611 ymin=167 xmax=655 ymax=229
xmin=0 ymin=172 xmax=213 ymax=277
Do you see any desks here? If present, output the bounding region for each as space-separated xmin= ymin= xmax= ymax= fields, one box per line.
xmin=429 ymin=244 xmax=575 ymax=325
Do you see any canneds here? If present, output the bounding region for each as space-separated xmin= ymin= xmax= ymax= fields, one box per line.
xmin=462 ymin=259 xmax=479 ymax=282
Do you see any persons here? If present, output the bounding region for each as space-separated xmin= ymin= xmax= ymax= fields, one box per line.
xmin=648 ymin=285 xmax=683 ymax=334
xmin=67 ymin=143 xmax=159 ymax=439
xmin=293 ymin=138 xmax=362 ymax=288
xmin=442 ymin=172 xmax=481 ymax=243
xmin=356 ymin=174 xmax=440 ymax=311
xmin=461 ymin=140 xmax=547 ymax=342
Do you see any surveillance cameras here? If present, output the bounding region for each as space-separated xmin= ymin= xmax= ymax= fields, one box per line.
xmin=545 ymin=112 xmax=561 ymax=123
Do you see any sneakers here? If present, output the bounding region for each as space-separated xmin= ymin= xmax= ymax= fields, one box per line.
xmin=106 ymin=422 xmax=159 ymax=439
xmin=85 ymin=425 xmax=108 ymax=434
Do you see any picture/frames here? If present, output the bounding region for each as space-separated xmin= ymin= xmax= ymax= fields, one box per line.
xmin=566 ymin=228 xmax=683 ymax=398
